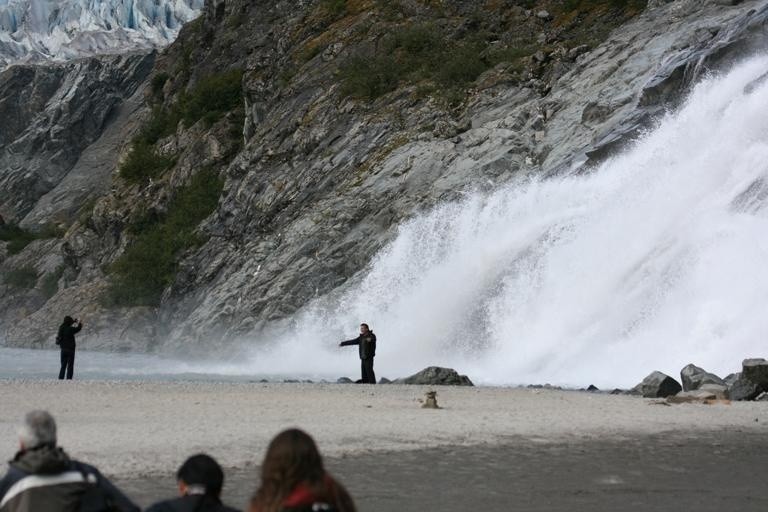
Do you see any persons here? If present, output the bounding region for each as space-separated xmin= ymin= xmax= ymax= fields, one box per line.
xmin=0 ymin=409 xmax=141 ymax=511
xmin=339 ymin=323 xmax=377 ymax=384
xmin=144 ymin=454 xmax=243 ymax=511
xmin=246 ymin=428 xmax=357 ymax=512
xmin=58 ymin=315 xmax=84 ymax=380
